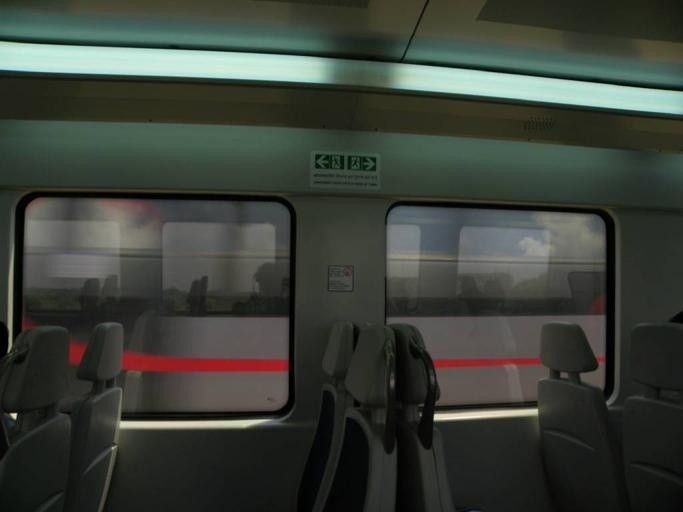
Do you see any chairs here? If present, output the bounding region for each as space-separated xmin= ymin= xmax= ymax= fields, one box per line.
xmin=623 ymin=323 xmax=683 ymax=512
xmin=72 ymin=278 xmax=100 ymax=312
xmin=298 ymin=321 xmax=455 ymax=512
xmin=65 ymin=322 xmax=123 ymax=512
xmin=187 ymin=279 xmax=201 ymax=314
xmin=0 ymin=325 xmax=73 ymax=512
xmin=101 ymin=275 xmax=120 ymax=312
xmin=200 ymin=276 xmax=208 ymax=314
xmin=538 ymin=322 xmax=630 ymax=512
